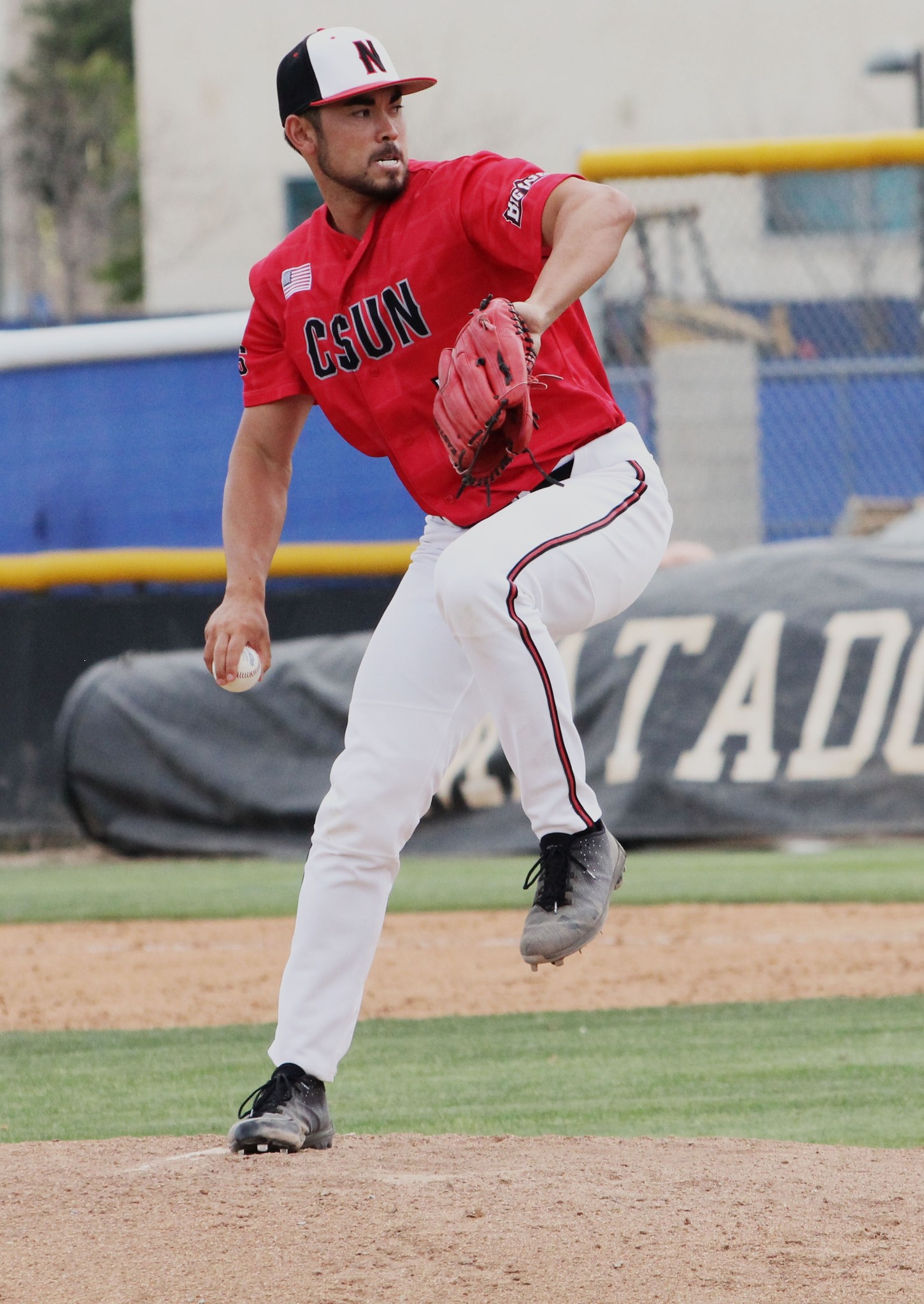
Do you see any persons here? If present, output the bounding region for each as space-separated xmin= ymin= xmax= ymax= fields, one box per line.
xmin=205 ymin=26 xmax=676 ymax=1153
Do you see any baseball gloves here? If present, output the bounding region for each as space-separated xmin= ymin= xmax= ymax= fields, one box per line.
xmin=430 ymin=293 xmax=564 ymax=509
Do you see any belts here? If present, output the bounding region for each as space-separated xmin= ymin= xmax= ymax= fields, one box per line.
xmin=459 ymin=458 xmax=575 ymax=529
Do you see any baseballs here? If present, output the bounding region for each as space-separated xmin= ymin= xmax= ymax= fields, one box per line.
xmin=210 ymin=645 xmax=263 ymax=694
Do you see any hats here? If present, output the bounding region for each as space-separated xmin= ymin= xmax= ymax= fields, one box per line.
xmin=277 ymin=28 xmax=438 ymax=127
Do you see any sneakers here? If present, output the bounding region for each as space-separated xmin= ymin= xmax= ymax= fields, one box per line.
xmin=228 ymin=1063 xmax=334 ymax=1154
xmin=520 ymin=821 xmax=627 ymax=971
